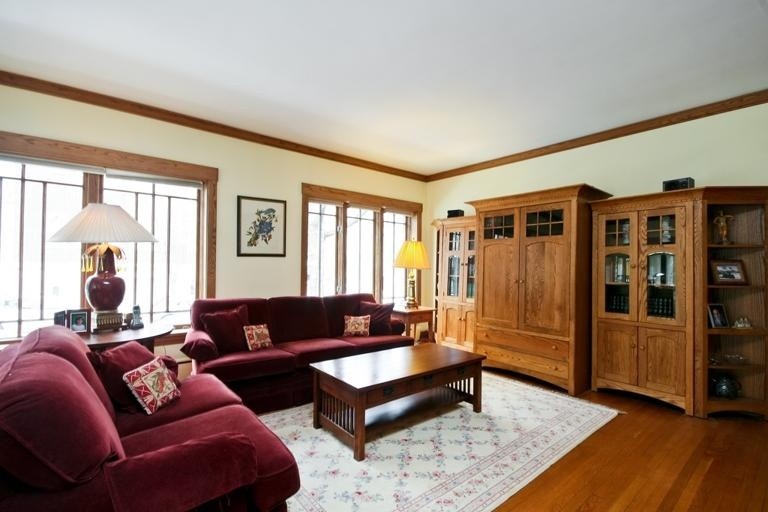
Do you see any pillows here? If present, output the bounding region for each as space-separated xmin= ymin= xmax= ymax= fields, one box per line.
xmin=343 ymin=314 xmax=371 ymax=337
xmin=360 ymin=300 xmax=395 ymax=335
xmin=242 ymin=324 xmax=274 ymax=352
xmin=86 ymin=339 xmax=157 ymax=413
xmin=199 ymin=304 xmax=251 ymax=354
xmin=123 ymin=355 xmax=181 ymax=414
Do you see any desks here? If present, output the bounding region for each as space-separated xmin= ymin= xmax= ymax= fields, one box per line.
xmin=392 ymin=304 xmax=438 ymax=343
xmin=79 ymin=322 xmax=175 ymax=356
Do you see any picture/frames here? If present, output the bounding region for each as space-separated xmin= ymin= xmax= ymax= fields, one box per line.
xmin=706 ymin=303 xmax=730 ymax=329
xmin=67 ymin=308 xmax=92 ymax=335
xmin=708 ymin=258 xmax=751 ymax=286
xmin=53 ymin=310 xmax=66 ymax=326
xmin=236 ymin=195 xmax=287 ymax=257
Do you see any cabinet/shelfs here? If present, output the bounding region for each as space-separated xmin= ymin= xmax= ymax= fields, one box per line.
xmin=467 ymin=182 xmax=614 ymax=396
xmin=694 ymin=185 xmax=768 ymax=421
xmin=585 ymin=186 xmax=695 ymax=416
xmin=431 ymin=216 xmax=475 ymax=353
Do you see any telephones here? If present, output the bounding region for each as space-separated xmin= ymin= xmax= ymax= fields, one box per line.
xmin=131 ymin=305 xmax=144 ymax=329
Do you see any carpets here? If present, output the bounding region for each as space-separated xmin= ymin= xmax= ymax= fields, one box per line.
xmin=256 ymin=368 xmax=628 ymax=511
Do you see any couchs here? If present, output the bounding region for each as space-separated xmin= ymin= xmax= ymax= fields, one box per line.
xmin=179 ymin=292 xmax=415 ymax=417
xmin=0 ymin=323 xmax=301 ymax=511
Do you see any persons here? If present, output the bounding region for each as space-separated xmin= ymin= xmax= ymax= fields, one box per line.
xmin=72 ymin=314 xmax=85 ymax=330
xmin=712 ymin=210 xmax=734 ymax=241
xmin=712 ymin=308 xmax=727 ymax=326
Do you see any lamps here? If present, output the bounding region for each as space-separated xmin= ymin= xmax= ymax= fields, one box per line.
xmin=391 ymin=237 xmax=433 ymax=310
xmin=48 ymin=203 xmax=159 ymax=331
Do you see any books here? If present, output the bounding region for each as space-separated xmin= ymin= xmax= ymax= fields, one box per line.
xmin=448 ymin=231 xmax=475 ymax=298
xmin=604 ymin=254 xmax=674 ymax=318
